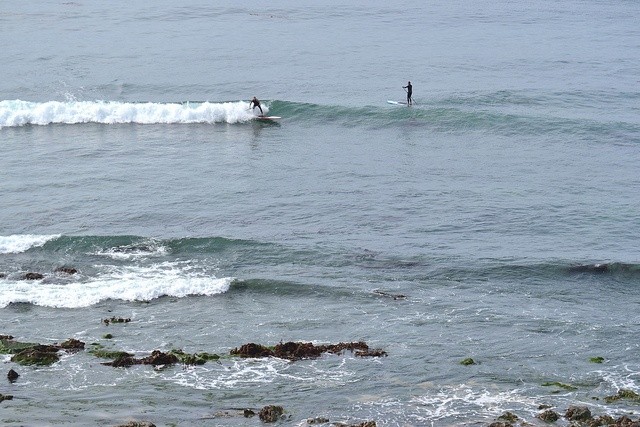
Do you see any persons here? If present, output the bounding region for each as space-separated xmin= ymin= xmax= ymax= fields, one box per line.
xmin=402 ymin=81 xmax=412 ymax=107
xmin=249 ymin=96 xmax=263 ymax=117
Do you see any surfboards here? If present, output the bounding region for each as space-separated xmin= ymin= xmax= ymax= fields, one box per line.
xmin=256 ymin=116 xmax=281 ymax=119
xmin=386 ymin=100 xmax=408 ymax=106
